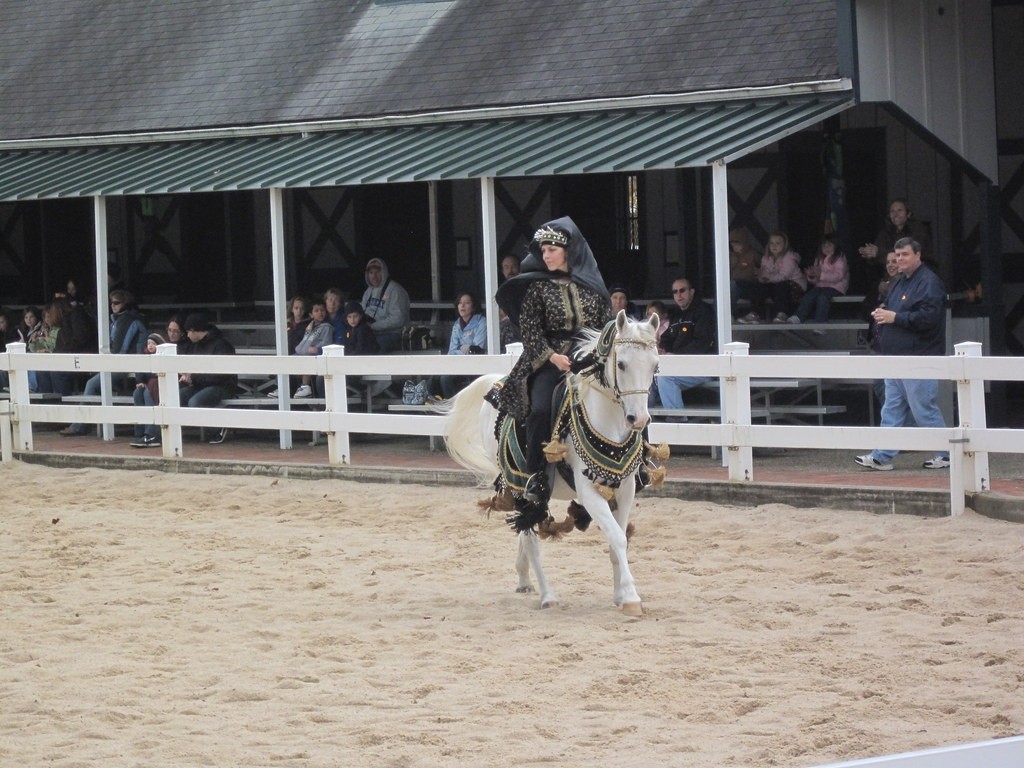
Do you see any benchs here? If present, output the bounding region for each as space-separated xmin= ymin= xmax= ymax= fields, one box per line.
xmin=0 ymin=294 xmax=954 ymax=460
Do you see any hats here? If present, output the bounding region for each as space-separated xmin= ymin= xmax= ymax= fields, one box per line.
xmin=608 ymin=280 xmax=630 ymax=298
xmin=146 ymin=328 xmax=167 ymax=344
xmin=367 ymin=261 xmax=380 ymax=268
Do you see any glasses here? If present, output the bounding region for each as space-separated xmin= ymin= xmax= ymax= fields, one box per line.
xmin=109 ymin=300 xmax=122 ymax=305
xmin=670 ymin=288 xmax=685 ymax=294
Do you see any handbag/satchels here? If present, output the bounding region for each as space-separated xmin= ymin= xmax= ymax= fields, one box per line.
xmin=402 ymin=325 xmax=432 ymax=351
xmin=402 ymin=379 xmax=430 ymax=405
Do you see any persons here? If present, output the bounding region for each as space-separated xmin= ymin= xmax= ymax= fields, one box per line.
xmin=425 ymin=253 xmax=718 ymax=423
xmin=495 ymin=215 xmax=651 ymax=501
xmin=267 ymin=258 xmax=410 ymax=398
xmin=854 ymin=237 xmax=950 ymax=471
xmin=730 ymin=197 xmax=931 ymax=337
xmin=0 ymin=262 xmax=238 ymax=448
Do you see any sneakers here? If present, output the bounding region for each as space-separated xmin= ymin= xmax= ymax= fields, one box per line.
xmin=208 ymin=428 xmax=227 ymax=443
xmin=267 ymin=389 xmax=278 ymax=398
xmin=855 ymin=453 xmax=894 ymax=471
xmin=129 ymin=435 xmax=162 ymax=446
xmin=923 ymin=456 xmax=950 ymax=469
xmin=293 ymin=384 xmax=313 ymax=399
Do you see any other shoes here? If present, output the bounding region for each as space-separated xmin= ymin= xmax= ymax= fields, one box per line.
xmin=773 ymin=312 xmax=786 ymax=323
xmin=738 ymin=312 xmax=760 ymax=325
xmin=812 ymin=329 xmax=828 ymax=335
xmin=59 ymin=425 xmax=87 ymax=435
xmin=786 ymin=315 xmax=801 ymax=323
xmin=524 ymin=471 xmax=550 ymax=495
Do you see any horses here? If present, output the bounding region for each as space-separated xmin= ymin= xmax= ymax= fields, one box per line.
xmin=424 ymin=308 xmax=661 ymax=616
